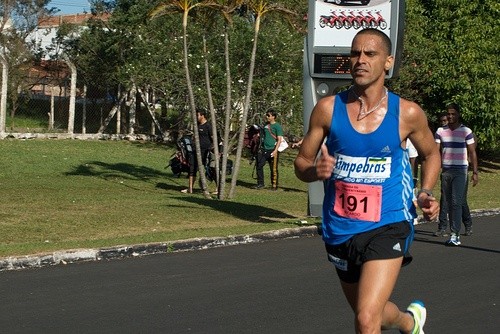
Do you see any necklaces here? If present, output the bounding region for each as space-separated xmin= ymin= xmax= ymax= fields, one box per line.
xmin=350 ymin=85 xmax=388 ymax=116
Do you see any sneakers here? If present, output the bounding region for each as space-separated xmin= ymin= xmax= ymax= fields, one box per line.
xmin=399 ymin=299 xmax=426 ymax=334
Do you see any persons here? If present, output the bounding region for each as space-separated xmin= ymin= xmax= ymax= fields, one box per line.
xmin=181 ymin=109 xmax=218 ymax=194
xmin=294 ymin=28 xmax=441 ymax=334
xmin=435 ymin=106 xmax=478 ymax=245
xmin=405 ymin=135 xmax=418 ymax=217
xmin=433 ymin=109 xmax=477 ymax=236
xmin=248 ymin=116 xmax=263 ymax=156
xmin=250 ymin=108 xmax=283 ymax=191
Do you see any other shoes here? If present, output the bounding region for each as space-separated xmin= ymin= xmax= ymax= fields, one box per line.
xmin=250 ymin=184 xmax=264 ymax=189
xmin=448 ymin=232 xmax=461 ymax=246
xmin=433 ymin=229 xmax=448 ymax=236
xmin=465 ymin=224 xmax=473 ymax=235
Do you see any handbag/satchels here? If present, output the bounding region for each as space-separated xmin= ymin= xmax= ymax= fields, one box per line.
xmin=205 ymin=167 xmax=216 ymax=182
xmin=275 ymin=138 xmax=288 ymax=152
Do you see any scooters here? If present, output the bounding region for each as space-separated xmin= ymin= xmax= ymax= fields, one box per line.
xmin=318 ymin=8 xmax=388 ymax=31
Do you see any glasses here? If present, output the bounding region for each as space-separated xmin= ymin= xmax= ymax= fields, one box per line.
xmin=266 ymin=114 xmax=270 ymax=117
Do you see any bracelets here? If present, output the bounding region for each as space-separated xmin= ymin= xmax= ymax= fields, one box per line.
xmin=473 ymin=171 xmax=477 ymax=176
xmin=417 ymin=189 xmax=433 ymax=196
